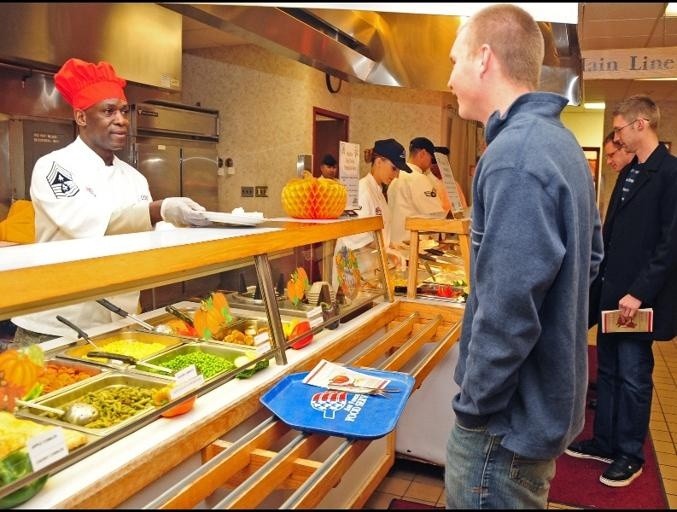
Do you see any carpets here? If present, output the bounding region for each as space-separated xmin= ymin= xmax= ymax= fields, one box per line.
xmin=548 ymin=343 xmax=669 ymax=512
xmin=387 ymin=498 xmax=447 ymax=512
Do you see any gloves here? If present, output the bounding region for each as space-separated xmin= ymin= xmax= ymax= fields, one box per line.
xmin=160 ymin=197 xmax=207 ymax=227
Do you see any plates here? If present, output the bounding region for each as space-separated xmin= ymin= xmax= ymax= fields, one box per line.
xmin=197 ymin=211 xmax=265 ymax=226
xmin=261 ymin=367 xmax=413 ymax=437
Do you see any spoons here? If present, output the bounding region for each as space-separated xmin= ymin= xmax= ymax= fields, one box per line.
xmin=55 ymin=315 xmax=98 ymax=351
xmin=86 ymin=351 xmax=171 ymax=376
xmin=165 ymin=304 xmax=195 ymax=330
xmin=101 ymin=300 xmax=159 ymax=331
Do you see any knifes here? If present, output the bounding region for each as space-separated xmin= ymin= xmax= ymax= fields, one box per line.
xmin=352 ymin=385 xmax=401 ymax=393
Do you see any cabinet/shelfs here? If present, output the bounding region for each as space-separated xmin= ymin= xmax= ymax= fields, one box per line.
xmin=361 ymin=211 xmax=473 ymax=467
xmin=0 ymin=215 xmax=465 ymax=512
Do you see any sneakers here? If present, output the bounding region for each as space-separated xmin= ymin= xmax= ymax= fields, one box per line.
xmin=562 ymin=441 xmax=608 ymax=461
xmin=601 ymin=455 xmax=644 ymax=487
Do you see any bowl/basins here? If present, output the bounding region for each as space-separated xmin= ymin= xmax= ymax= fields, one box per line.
xmin=7 ymin=359 xmax=105 ymax=401
xmin=210 ymin=318 xmax=297 ymax=348
xmin=142 ymin=312 xmax=239 ymax=339
xmin=17 ymin=370 xmax=174 ymax=434
xmin=307 ymin=282 xmax=336 ymax=305
xmin=128 ymin=343 xmax=247 ymax=383
xmin=61 ymin=330 xmax=183 ymax=368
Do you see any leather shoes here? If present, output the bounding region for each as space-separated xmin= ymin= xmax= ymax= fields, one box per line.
xmin=588 ymin=382 xmax=598 ymax=391
xmin=588 ymin=399 xmax=599 ymax=409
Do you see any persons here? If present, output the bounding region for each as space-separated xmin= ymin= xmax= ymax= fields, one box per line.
xmin=562 ymin=94 xmax=677 ymax=486
xmin=387 ymin=137 xmax=443 ymax=249
xmin=428 ymin=145 xmax=469 ymax=224
xmin=445 ymin=5 xmax=605 ymax=512
xmin=319 ymin=155 xmax=340 ymax=180
xmin=585 ymin=129 xmax=639 ymax=411
xmin=332 ymin=139 xmax=413 ymax=293
xmin=11 ymin=59 xmax=214 ymax=348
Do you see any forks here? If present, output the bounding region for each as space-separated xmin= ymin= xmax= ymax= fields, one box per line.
xmin=327 ymin=386 xmax=392 ymax=400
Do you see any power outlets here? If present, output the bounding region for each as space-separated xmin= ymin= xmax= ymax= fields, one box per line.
xmin=228 ymin=168 xmax=235 ymax=174
xmin=218 ymin=168 xmax=225 ymax=175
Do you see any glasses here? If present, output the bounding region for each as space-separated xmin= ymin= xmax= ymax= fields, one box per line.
xmin=603 ymin=146 xmax=621 ymax=158
xmin=612 ymin=118 xmax=649 ymax=135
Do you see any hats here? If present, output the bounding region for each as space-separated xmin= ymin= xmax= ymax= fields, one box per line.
xmin=51 ymin=56 xmax=127 ymax=114
xmin=372 ymin=138 xmax=410 ymax=175
xmin=411 ymin=136 xmax=438 ymax=165
